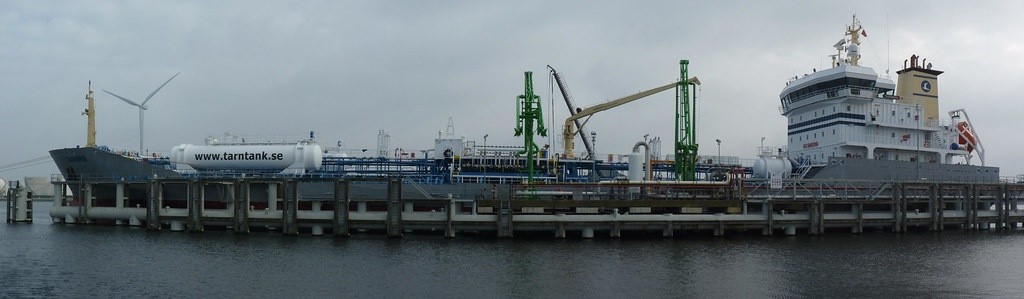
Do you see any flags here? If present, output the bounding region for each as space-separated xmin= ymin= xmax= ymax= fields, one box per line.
xmin=861 ymin=30 xmax=867 ymax=37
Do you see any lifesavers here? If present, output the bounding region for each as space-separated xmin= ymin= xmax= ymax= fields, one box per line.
xmin=902 ymin=134 xmax=908 ymax=141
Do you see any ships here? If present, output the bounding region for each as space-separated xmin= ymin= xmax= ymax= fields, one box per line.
xmin=50 ymin=15 xmax=1007 ymax=211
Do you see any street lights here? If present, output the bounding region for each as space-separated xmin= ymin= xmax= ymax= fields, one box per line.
xmin=591 ymin=131 xmax=596 ymax=200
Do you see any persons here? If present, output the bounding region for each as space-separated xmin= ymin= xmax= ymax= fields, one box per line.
xmin=958 ymin=162 xmax=968 ymax=165
xmin=847 ymin=154 xmax=861 ymax=159
xmin=798 ymin=152 xmax=811 ymax=164
xmin=911 ymin=157 xmax=915 ymax=161
